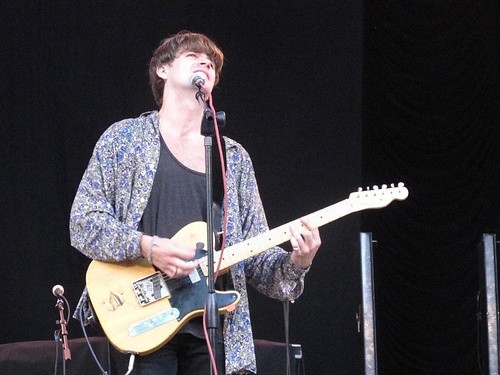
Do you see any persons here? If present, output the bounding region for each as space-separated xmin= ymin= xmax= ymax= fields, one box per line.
xmin=69 ymin=32 xmax=321 ymax=375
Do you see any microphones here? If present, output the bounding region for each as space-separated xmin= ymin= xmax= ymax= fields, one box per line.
xmin=52 ymin=284 xmax=64 ymax=297
xmin=190 ymin=72 xmax=204 ymax=87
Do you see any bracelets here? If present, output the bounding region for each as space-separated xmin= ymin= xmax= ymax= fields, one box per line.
xmin=147 ymin=235 xmax=158 ymax=264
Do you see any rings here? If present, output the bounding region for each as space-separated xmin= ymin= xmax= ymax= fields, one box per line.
xmin=174 ymin=267 xmax=178 ymax=275
xmin=293 ymin=247 xmax=301 ymax=254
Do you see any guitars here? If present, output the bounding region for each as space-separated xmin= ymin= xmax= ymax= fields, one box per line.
xmin=85 ymin=180 xmax=409 ymax=356
